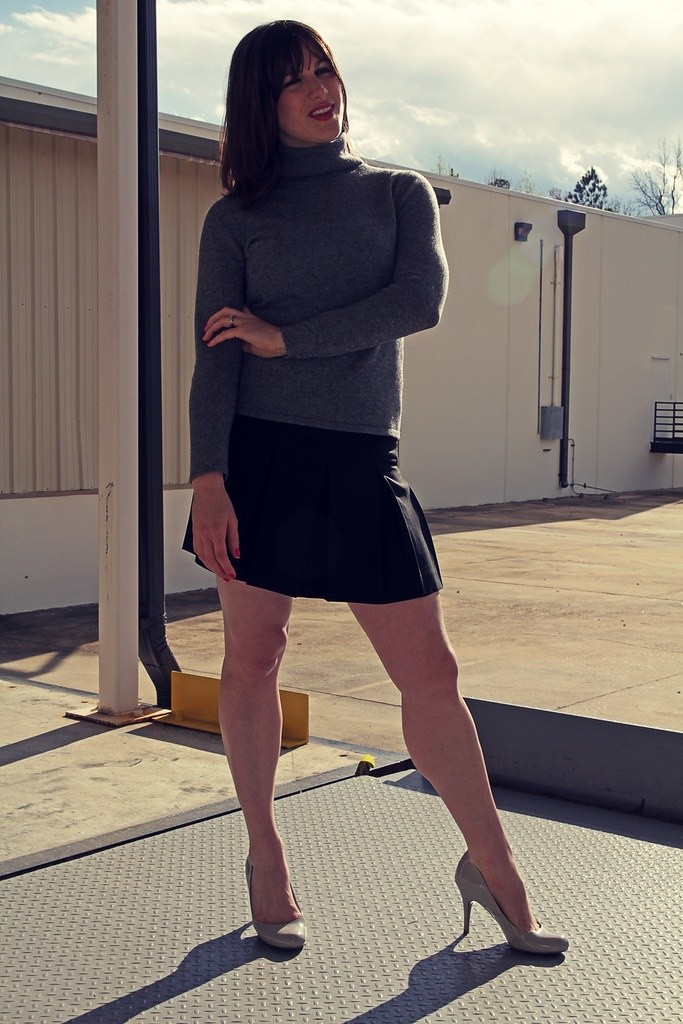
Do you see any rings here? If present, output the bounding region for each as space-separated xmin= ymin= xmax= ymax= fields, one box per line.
xmin=230 ymin=316 xmax=235 ymax=326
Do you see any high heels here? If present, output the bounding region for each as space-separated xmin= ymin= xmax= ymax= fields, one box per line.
xmin=455 ymin=850 xmax=570 ymax=953
xmin=245 ymin=854 xmax=306 ymax=949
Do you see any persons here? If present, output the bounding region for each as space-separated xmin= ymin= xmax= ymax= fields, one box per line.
xmin=182 ymin=21 xmax=571 ymax=952
